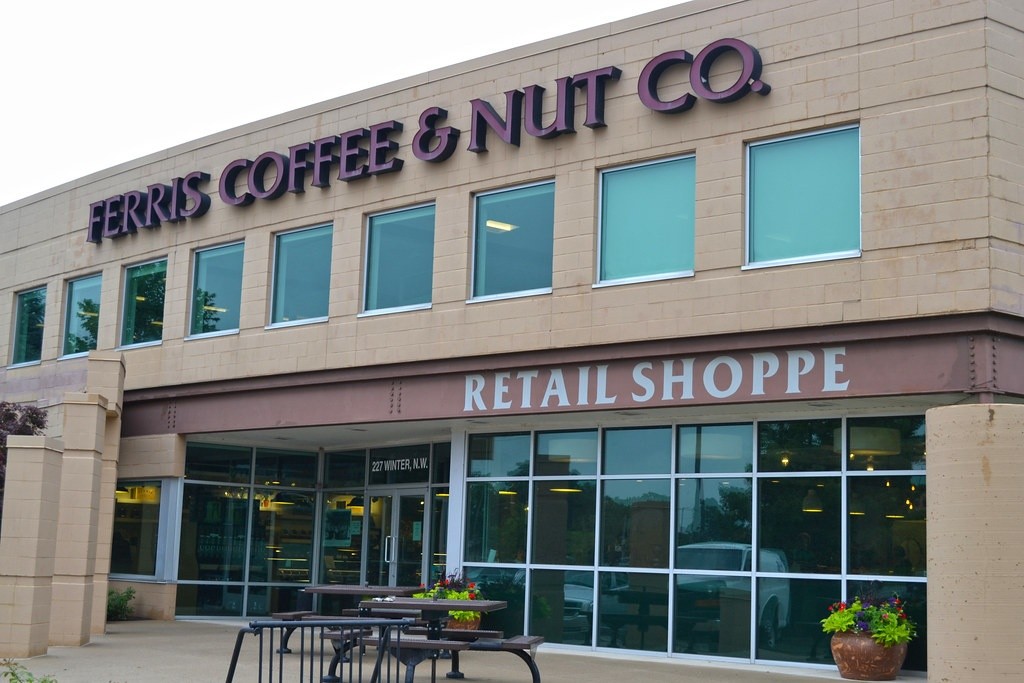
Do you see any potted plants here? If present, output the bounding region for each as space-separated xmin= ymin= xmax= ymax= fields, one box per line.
xmin=259 ymin=482 xmax=270 ymax=507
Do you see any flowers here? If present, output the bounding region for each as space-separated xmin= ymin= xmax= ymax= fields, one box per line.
xmin=413 ymin=571 xmax=490 ymax=622
xmin=478 ymin=575 xmax=526 ymax=605
xmin=820 ymin=586 xmax=917 ymax=648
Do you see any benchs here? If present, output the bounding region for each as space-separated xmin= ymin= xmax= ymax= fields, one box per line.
xmin=358 ymin=636 xmax=471 ymax=652
xmin=579 ymin=611 xmax=706 ymax=623
xmin=272 ymin=611 xmax=316 ymax=621
xmin=320 ymin=629 xmax=374 ymax=640
xmin=500 ymin=635 xmax=544 ymax=653
xmin=303 ymin=615 xmax=384 ymax=622
xmin=343 ymin=609 xmax=421 ymax=619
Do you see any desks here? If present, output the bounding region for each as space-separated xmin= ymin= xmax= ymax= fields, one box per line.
xmin=276 ymin=584 xmax=434 ymax=663
xmin=577 ymin=586 xmax=703 ymax=654
xmin=321 ymin=597 xmax=540 ymax=683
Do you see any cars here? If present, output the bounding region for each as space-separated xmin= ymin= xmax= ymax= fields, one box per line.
xmin=516 ymin=543 xmax=791 ymax=650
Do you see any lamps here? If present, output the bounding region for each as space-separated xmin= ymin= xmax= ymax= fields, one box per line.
xmin=850 ymin=492 xmax=866 ymax=515
xmin=346 ymin=496 xmax=365 ymax=509
xmin=884 ymin=491 xmax=905 ymax=518
xmin=271 ymin=491 xmax=295 ymax=505
xmin=866 ymin=456 xmax=874 ymax=471
xmin=803 ymin=482 xmax=823 ymax=512
xmin=550 ymin=473 xmax=584 ymax=492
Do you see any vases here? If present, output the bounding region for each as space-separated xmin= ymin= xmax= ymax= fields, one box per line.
xmin=481 ymin=597 xmax=527 ymax=638
xmin=830 ymin=631 xmax=905 ymax=681
xmin=440 ymin=616 xmax=480 ymax=642
